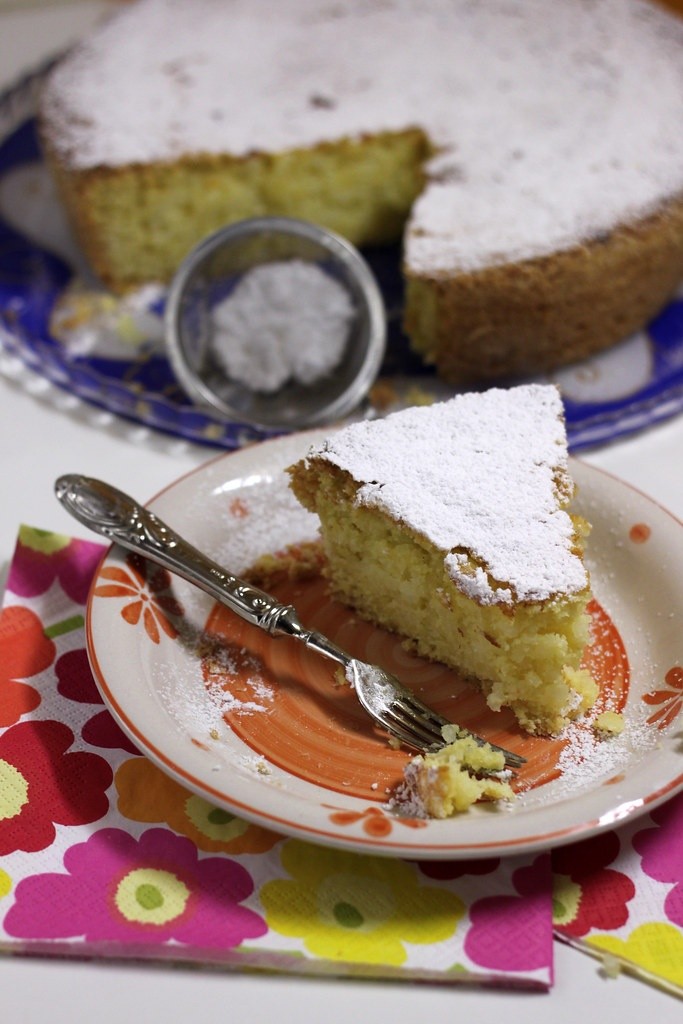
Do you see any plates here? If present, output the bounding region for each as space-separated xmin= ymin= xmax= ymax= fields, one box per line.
xmin=88 ymin=426 xmax=683 ymax=858
xmin=0 ymin=38 xmax=683 ymax=456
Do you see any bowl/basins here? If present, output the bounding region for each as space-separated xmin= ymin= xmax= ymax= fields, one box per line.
xmin=164 ymin=216 xmax=386 ymax=436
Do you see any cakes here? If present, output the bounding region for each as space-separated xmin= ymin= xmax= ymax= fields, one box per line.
xmin=281 ymin=383 xmax=593 ymax=735
xmin=39 ymin=0 xmax=683 ymax=385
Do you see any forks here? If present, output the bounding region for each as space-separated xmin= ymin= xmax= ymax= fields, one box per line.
xmin=55 ymin=472 xmax=531 ymax=783
